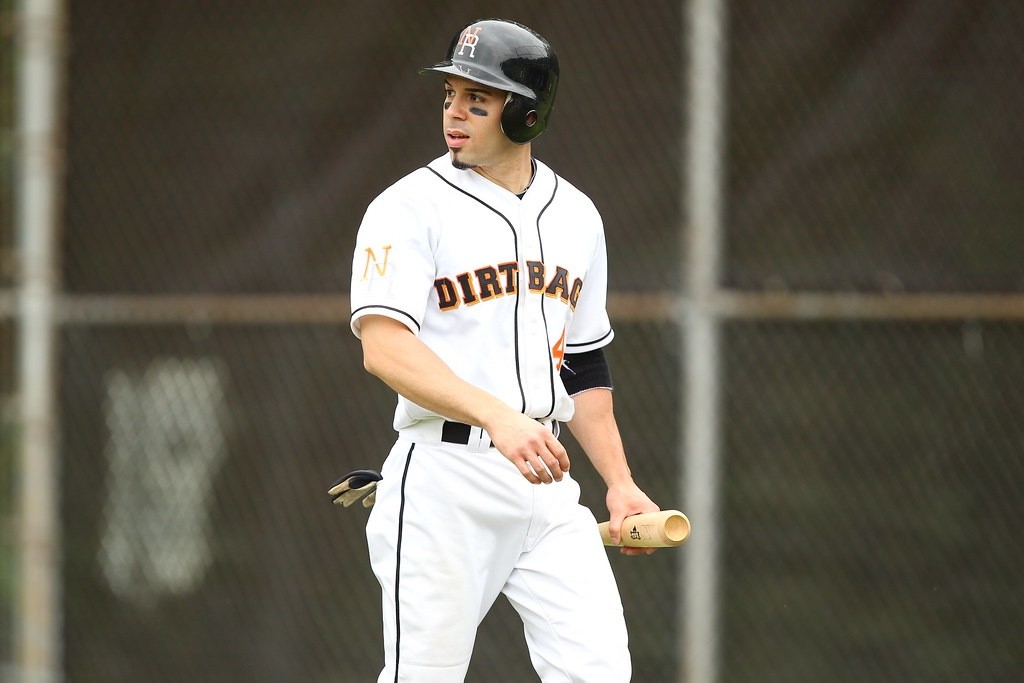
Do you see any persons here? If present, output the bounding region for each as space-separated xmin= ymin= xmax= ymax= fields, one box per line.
xmin=350 ymin=20 xmax=660 ymax=682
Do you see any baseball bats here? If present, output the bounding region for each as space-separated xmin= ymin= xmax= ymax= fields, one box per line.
xmin=597 ymin=510 xmax=690 ymax=549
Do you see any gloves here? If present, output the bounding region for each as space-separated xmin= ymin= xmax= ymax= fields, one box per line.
xmin=327 ymin=470 xmax=382 ymax=508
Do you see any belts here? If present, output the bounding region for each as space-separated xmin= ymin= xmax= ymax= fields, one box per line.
xmin=441 ymin=420 xmax=494 ymax=447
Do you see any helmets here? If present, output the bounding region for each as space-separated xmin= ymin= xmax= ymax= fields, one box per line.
xmin=417 ymin=18 xmax=560 ymax=145
